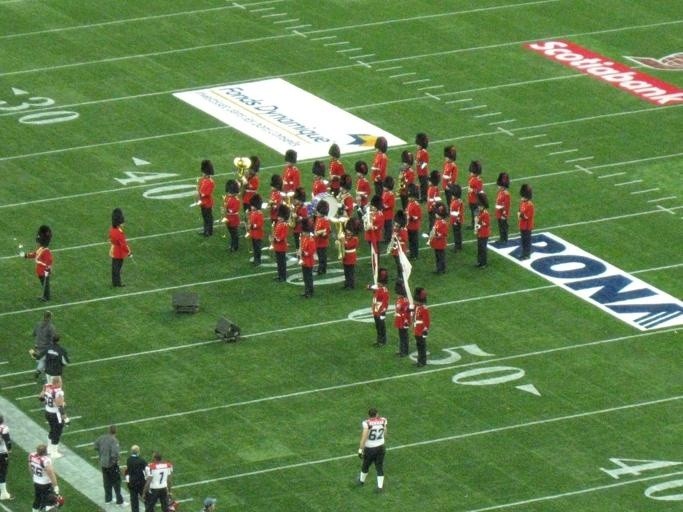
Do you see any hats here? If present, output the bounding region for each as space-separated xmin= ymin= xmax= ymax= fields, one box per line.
xmin=112 ymin=207 xmax=124 ymax=227
xmin=35 ymin=226 xmax=51 ymax=245
xmin=204 ymin=498 xmax=216 ymax=506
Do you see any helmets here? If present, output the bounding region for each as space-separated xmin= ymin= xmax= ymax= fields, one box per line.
xmin=168 ymin=501 xmax=178 ymax=511
xmin=47 ymin=493 xmax=64 ymax=505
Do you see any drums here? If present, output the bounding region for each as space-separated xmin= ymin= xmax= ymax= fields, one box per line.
xmin=311 ymin=192 xmax=340 ymax=218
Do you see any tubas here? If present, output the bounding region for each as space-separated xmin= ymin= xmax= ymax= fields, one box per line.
xmin=362 ymin=204 xmax=377 ymax=230
xmin=280 ymin=191 xmax=296 ymax=228
xmin=234 ymin=157 xmax=251 ymax=198
xmin=329 ymin=217 xmax=350 ymax=264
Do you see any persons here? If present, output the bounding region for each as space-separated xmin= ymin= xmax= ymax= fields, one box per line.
xmin=23 ymin=310 xmax=54 ymax=362
xmin=371 ymin=132 xmax=535 ymax=276
xmin=409 ymin=300 xmax=429 ymax=368
xmin=369 ymin=280 xmax=389 ymax=348
xmin=356 ymin=406 xmax=386 ymax=492
xmin=109 ymin=221 xmax=131 ymax=287
xmin=16 ymin=240 xmax=54 ymax=302
xmin=0 ymin=372 xmax=221 ymax=512
xmin=27 ymin=333 xmax=69 ymax=400
xmin=192 ymin=150 xmax=409 ymax=300
xmin=390 ymin=293 xmax=411 ymax=357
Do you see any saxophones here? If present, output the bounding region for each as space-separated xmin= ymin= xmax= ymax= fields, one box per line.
xmin=396 ymin=169 xmax=406 ymax=196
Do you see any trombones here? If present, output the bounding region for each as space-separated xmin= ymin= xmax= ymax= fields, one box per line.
xmin=297 ymin=232 xmax=304 ymax=283
xmin=218 ymin=194 xmax=228 ymax=237
xmin=268 ymin=220 xmax=274 ymax=263
xmin=244 ymin=209 xmax=255 ymax=253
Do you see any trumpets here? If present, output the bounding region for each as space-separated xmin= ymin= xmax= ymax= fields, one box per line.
xmin=424 ymin=224 xmax=436 ymax=246
xmin=474 ymin=212 xmax=482 ymax=236
xmin=386 ymin=232 xmax=399 ymax=255
